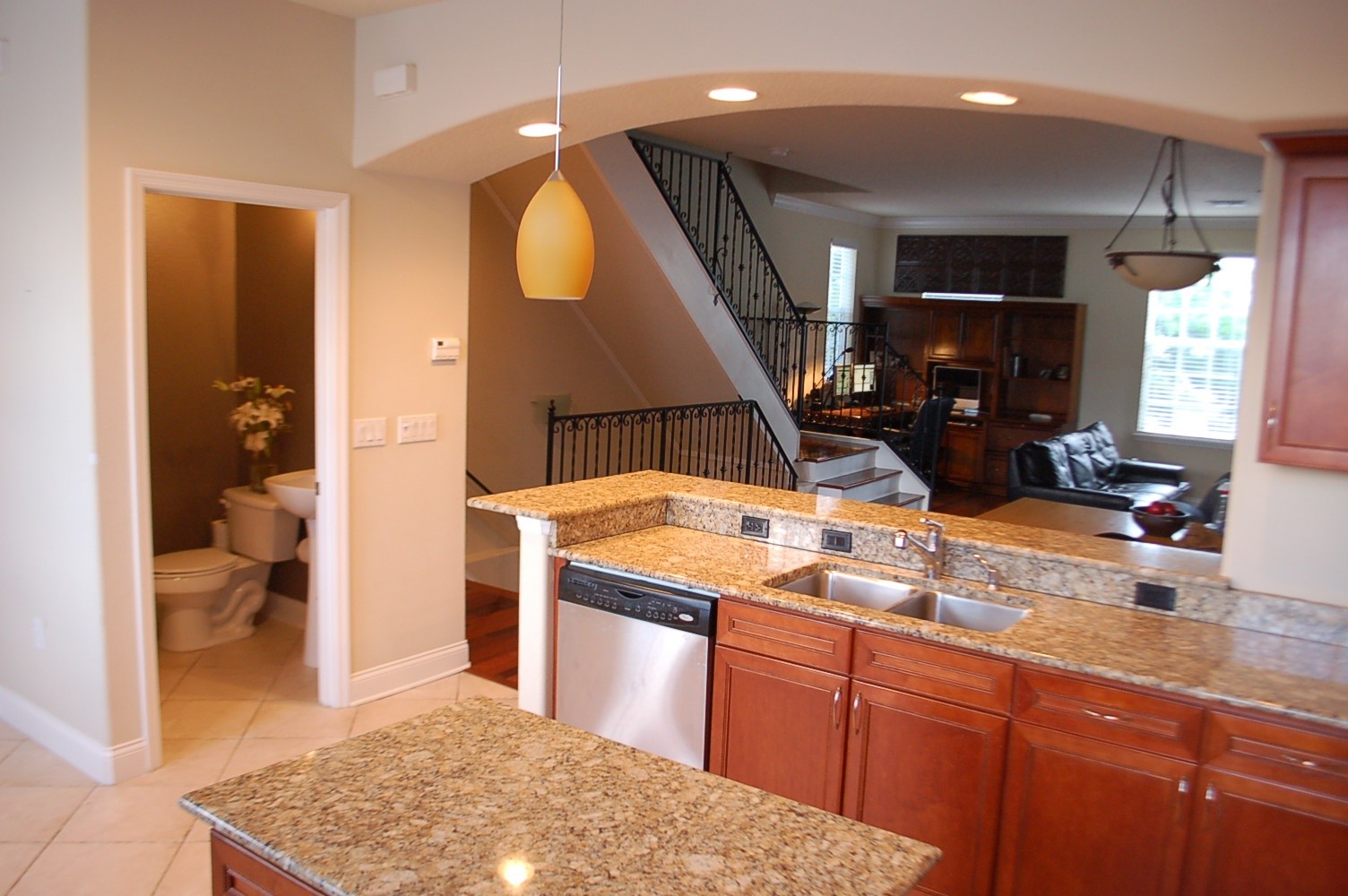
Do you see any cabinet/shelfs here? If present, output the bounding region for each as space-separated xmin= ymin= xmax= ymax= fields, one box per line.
xmin=707 ymin=555 xmax=1024 ymax=896
xmin=856 ymin=291 xmax=1090 ymax=430
xmin=931 ymin=411 xmax=983 ymax=488
xmin=986 ymin=586 xmax=1348 ymax=896
xmin=1254 ymin=126 xmax=1347 ymax=472
xmin=980 ymin=417 xmax=1079 ymax=497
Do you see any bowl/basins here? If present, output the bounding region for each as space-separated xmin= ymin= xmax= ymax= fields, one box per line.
xmin=1129 ymin=503 xmax=1191 ymax=537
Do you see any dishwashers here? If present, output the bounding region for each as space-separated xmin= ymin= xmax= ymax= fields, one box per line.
xmin=555 ymin=559 xmax=720 ymax=777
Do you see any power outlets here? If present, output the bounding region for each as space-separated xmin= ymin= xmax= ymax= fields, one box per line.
xmin=741 ymin=515 xmax=769 ymax=538
xmin=821 ymin=529 xmax=853 ymax=552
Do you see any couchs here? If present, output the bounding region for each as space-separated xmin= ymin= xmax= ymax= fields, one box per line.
xmin=1005 ymin=420 xmax=1193 ymax=512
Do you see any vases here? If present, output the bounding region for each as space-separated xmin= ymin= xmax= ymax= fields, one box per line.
xmin=247 ymin=448 xmax=281 ymax=495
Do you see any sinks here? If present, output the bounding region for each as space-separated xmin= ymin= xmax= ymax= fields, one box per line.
xmin=263 ymin=467 xmax=315 ymax=519
xmin=758 ymin=561 xmax=926 ymax=614
xmin=880 ymin=587 xmax=1032 ymax=636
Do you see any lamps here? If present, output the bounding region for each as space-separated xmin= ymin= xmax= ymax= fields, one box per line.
xmin=1102 ymin=133 xmax=1223 ymax=292
xmin=513 ymin=2 xmax=597 ymax=301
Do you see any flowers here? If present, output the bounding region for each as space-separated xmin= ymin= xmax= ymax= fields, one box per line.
xmin=212 ymin=374 xmax=296 ymax=458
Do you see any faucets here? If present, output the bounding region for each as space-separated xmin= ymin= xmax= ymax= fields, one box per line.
xmin=891 ymin=516 xmax=947 ymax=578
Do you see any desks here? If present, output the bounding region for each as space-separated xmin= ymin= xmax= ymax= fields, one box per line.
xmin=180 ymin=694 xmax=944 ymax=895
xmin=799 ymin=400 xmax=921 ymax=439
xmin=967 ymin=498 xmax=1224 ymax=554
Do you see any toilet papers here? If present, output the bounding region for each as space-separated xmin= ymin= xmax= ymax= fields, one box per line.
xmin=211 ymin=518 xmax=232 ymax=550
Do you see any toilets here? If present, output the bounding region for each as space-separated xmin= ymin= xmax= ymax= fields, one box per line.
xmin=152 ymin=484 xmax=301 ymax=653
